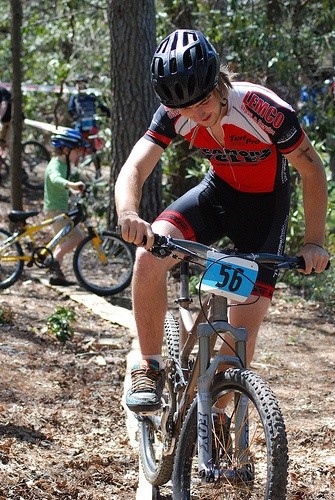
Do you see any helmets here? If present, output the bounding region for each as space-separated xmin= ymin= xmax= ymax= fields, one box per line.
xmin=150 ymin=29 xmax=221 ymax=108
xmin=51 ymin=130 xmax=82 ymax=150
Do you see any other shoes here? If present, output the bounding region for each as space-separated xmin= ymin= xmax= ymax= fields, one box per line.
xmin=49 ymin=276 xmax=77 ymax=287
xmin=44 ymin=257 xmax=64 ymax=278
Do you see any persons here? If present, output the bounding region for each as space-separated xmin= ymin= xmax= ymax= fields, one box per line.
xmin=113 ymin=30 xmax=331 ymax=489
xmin=62 ymin=76 xmax=112 ymax=180
xmin=41 ymin=129 xmax=89 ymax=287
xmin=0 ymin=86 xmax=16 ymax=153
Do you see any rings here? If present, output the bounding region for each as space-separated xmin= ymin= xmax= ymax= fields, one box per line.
xmin=313 ymin=266 xmax=317 ymax=268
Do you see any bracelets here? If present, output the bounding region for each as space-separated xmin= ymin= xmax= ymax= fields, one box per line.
xmin=119 ymin=211 xmax=138 ymax=217
xmin=303 ymin=242 xmax=326 ymax=250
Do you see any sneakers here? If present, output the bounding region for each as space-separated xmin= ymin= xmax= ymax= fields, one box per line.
xmin=211 ymin=413 xmax=233 ymax=467
xmin=126 ymin=359 xmax=166 ymax=410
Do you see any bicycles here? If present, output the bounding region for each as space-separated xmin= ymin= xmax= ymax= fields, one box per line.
xmin=0 ymin=184 xmax=135 ymax=296
xmin=103 ymin=225 xmax=331 ymax=499
xmin=0 ymin=131 xmax=104 ymax=181
